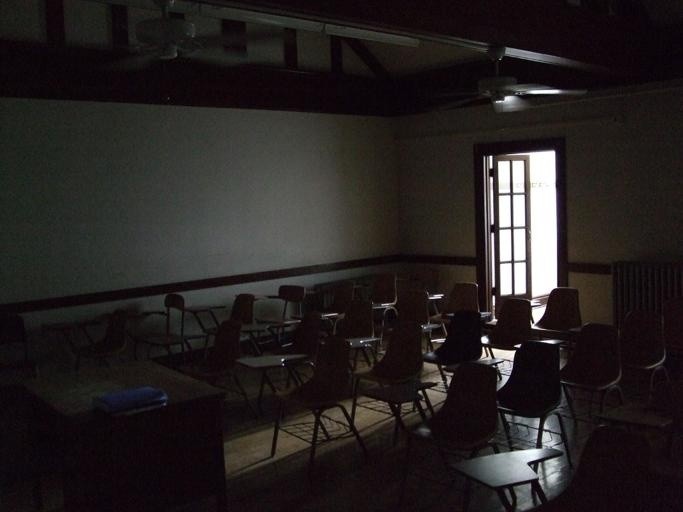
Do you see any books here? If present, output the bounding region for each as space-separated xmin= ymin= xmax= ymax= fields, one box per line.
xmin=90 ymin=384 xmax=168 ymax=416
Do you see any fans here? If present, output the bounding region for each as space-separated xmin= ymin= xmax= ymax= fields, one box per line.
xmin=50 ymin=0 xmax=301 ymax=70
xmin=436 ymin=42 xmax=591 ymax=111
